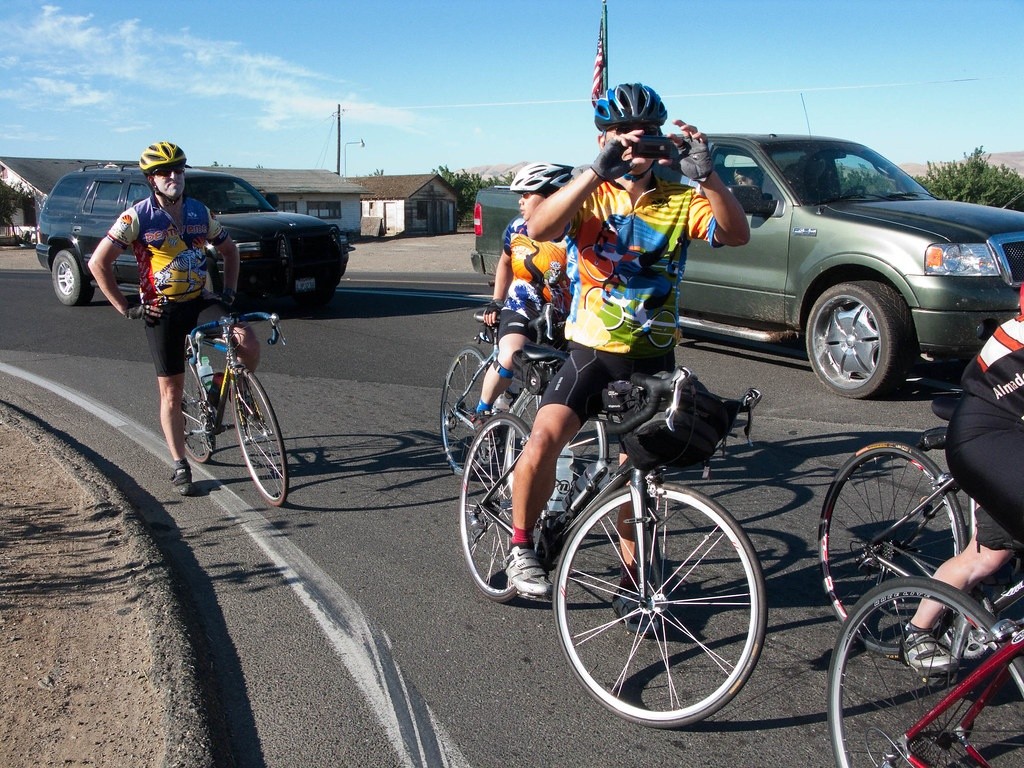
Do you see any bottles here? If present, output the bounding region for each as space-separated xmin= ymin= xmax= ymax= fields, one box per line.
xmin=561 ymin=457 xmax=613 ymax=517
xmin=198 ymin=356 xmax=224 ymax=409
xmin=505 ymin=380 xmax=522 ymax=407
xmin=546 ymin=441 xmax=572 ymax=512
xmin=491 ymin=390 xmax=513 ymax=416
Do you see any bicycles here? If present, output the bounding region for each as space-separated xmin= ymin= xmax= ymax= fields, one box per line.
xmin=437 ymin=303 xmax=608 ymax=530
xmin=455 ymin=342 xmax=769 ymax=731
xmin=830 ymin=576 xmax=1023 ymax=768
xmin=179 ymin=313 xmax=290 ymax=506
xmin=816 ymin=397 xmax=1024 ymax=679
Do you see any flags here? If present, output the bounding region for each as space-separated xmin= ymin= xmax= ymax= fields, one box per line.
xmin=591 ymin=13 xmax=607 ymax=147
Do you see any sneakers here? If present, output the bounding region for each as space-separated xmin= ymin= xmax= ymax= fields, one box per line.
xmin=502 ymin=545 xmax=552 ymax=596
xmin=611 ymin=588 xmax=659 ymax=636
xmin=471 ymin=409 xmax=495 ymax=436
xmin=169 ymin=458 xmax=195 ymax=495
xmin=899 ymin=624 xmax=958 ymax=677
xmin=229 ymin=388 xmax=263 ymax=430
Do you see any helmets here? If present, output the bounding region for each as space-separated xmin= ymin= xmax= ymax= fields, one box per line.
xmin=510 ymin=163 xmax=574 ymax=196
xmin=139 ymin=143 xmax=186 ymax=175
xmin=595 ymin=84 xmax=667 ymax=131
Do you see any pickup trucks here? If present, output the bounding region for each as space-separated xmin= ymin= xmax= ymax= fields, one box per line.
xmin=467 ymin=130 xmax=1024 ymax=400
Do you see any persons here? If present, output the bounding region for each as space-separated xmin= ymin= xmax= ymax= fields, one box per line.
xmin=897 ymin=316 xmax=1024 ymax=678
xmin=503 ymin=83 xmax=750 ymax=638
xmin=725 ymin=165 xmax=774 ymax=201
xmin=471 ymin=162 xmax=573 ymax=440
xmin=87 ymin=142 xmax=262 ymax=495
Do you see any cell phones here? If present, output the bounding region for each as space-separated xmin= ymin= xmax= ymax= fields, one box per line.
xmin=632 ymin=135 xmax=673 ymax=159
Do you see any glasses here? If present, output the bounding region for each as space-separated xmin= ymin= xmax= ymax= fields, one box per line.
xmin=150 ymin=166 xmax=185 ymax=177
xmin=606 ymin=123 xmax=659 ymax=136
xmin=520 ymin=191 xmax=542 ymax=199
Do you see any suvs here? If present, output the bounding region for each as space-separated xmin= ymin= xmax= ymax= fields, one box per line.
xmin=36 ymin=163 xmax=358 ymax=309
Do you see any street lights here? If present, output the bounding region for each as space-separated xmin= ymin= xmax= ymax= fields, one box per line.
xmin=344 ymin=139 xmax=366 ymax=180
